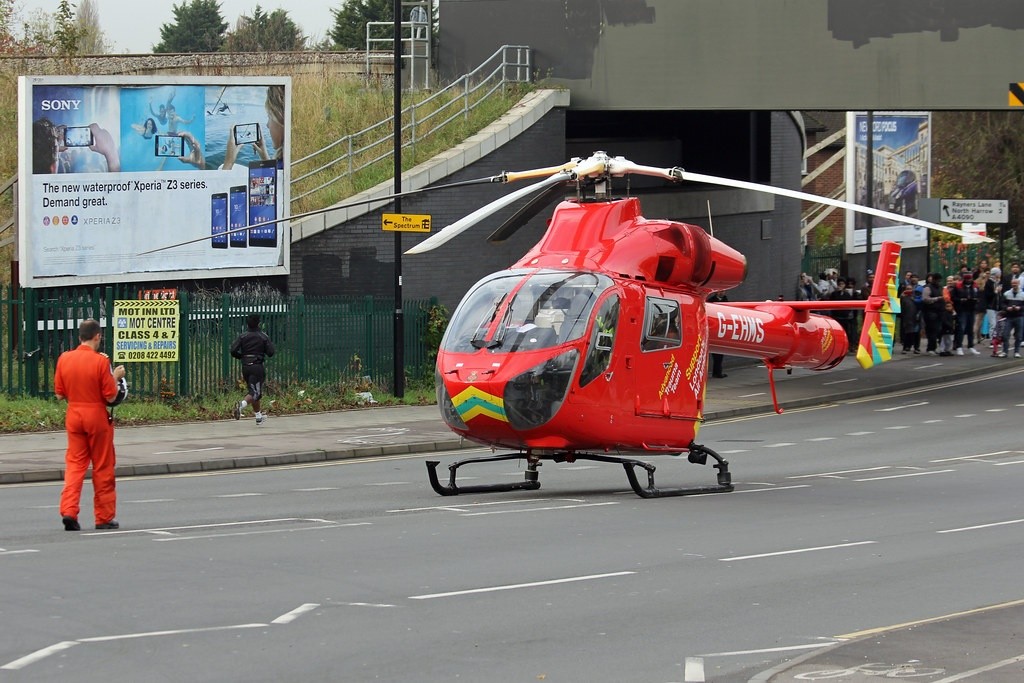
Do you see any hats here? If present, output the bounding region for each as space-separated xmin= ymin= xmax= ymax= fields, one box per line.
xmin=962 ymin=271 xmax=974 ymax=278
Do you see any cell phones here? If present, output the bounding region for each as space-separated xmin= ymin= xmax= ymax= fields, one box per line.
xmin=230 ymin=185 xmax=247 ymax=247
xmin=212 ymin=193 xmax=228 ymax=248
xmin=248 ymin=159 xmax=278 ymax=253
xmin=64 ymin=126 xmax=93 ymax=147
xmin=155 ymin=135 xmax=185 ymax=157
xmin=234 ymin=123 xmax=260 ymax=144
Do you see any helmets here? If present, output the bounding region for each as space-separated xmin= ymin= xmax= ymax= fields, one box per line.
xmin=106 ymin=376 xmax=129 ymax=407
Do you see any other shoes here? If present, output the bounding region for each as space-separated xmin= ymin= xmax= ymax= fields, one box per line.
xmin=1014 ymin=352 xmax=1020 ymax=357
xmin=713 ymin=374 xmax=724 ymax=378
xmin=906 ymin=347 xmax=910 ymax=351
xmin=989 ymin=345 xmax=994 ymax=348
xmin=913 ymin=349 xmax=920 ymax=353
xmin=999 ymin=352 xmax=1007 ymax=357
xmin=902 ymin=350 xmax=906 ymax=354
xmin=927 ymin=350 xmax=938 ymax=357
xmin=95 ymin=520 xmax=119 ymax=529
xmin=62 ymin=516 xmax=80 ymax=531
xmin=998 ymin=344 xmax=1002 ymax=347
xmin=939 ymin=351 xmax=954 ymax=357
xmin=991 ymin=351 xmax=998 ymax=356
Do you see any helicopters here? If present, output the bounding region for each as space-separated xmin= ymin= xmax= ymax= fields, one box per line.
xmin=137 ymin=149 xmax=996 ymax=501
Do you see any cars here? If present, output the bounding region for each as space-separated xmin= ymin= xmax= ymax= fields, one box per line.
xmin=888 ymin=169 xmax=919 ymax=215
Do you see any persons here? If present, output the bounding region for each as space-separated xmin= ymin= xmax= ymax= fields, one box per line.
xmin=231 ymin=315 xmax=274 ymax=426
xmin=132 ymin=89 xmax=205 ymax=171
xmin=54 ymin=320 xmax=125 ymax=531
xmin=800 ymin=260 xmax=1024 ymax=359
xmin=215 ymin=85 xmax=285 ymax=170
xmin=32 ymin=117 xmax=120 ymax=174
xmin=709 ymin=289 xmax=728 ymax=378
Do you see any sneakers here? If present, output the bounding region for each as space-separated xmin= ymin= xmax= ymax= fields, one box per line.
xmin=256 ymin=415 xmax=268 ymax=425
xmin=968 ymin=348 xmax=981 ymax=355
xmin=956 ymin=347 xmax=964 ymax=355
xmin=234 ymin=401 xmax=242 ymax=421
